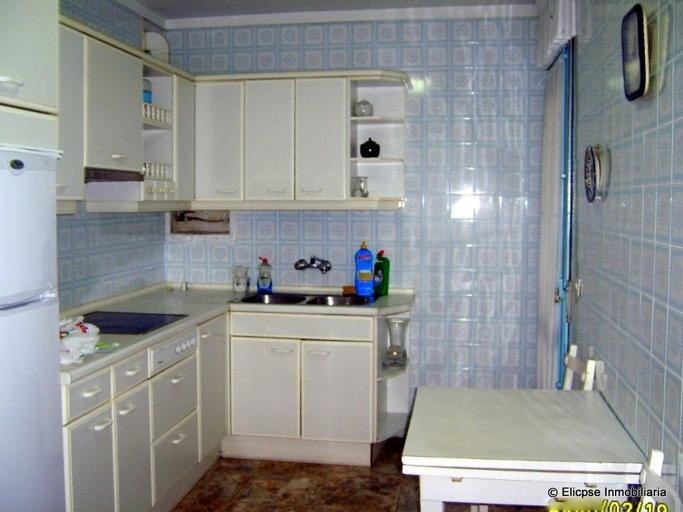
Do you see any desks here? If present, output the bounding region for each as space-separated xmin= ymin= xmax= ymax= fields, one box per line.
xmin=402 ymin=386 xmax=644 ymax=512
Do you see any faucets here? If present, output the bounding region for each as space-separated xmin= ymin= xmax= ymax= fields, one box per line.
xmin=319 ymin=260 xmax=333 ymax=274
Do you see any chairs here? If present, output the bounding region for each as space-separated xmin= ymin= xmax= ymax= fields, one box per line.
xmin=479 ymin=344 xmax=596 ymax=512
xmin=639 ymin=448 xmax=683 ymax=512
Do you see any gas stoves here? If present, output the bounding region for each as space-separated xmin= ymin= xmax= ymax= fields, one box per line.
xmin=79 ymin=312 xmax=187 ymax=337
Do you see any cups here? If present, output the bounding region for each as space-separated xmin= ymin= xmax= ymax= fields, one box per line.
xmin=142 ymin=103 xmax=171 ymax=178
xmin=351 ymin=175 xmax=370 ymax=197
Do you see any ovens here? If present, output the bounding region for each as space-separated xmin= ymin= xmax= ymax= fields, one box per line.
xmin=143 ymin=354 xmax=201 ymax=511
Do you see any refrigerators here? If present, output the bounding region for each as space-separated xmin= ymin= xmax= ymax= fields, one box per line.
xmin=0 ymin=142 xmax=71 ymax=512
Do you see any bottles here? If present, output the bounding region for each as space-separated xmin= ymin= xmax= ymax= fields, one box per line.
xmin=359 ymin=135 xmax=380 ymax=159
xmin=384 ymin=316 xmax=410 ymax=367
xmin=355 ymin=99 xmax=374 ymax=116
xmin=231 ymin=264 xmax=250 ymax=295
xmin=256 ymin=256 xmax=274 ymax=295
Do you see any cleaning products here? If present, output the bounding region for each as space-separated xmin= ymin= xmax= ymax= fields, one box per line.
xmin=375 ymin=250 xmax=389 ymax=296
xmin=257 ymin=257 xmax=273 ymax=293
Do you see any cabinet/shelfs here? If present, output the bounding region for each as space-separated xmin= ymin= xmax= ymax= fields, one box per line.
xmin=63 ymin=313 xmax=227 ymax=512
xmin=231 ymin=337 xmax=373 ymax=444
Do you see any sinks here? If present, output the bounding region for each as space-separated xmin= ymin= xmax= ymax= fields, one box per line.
xmin=227 ymin=292 xmax=305 ymax=305
xmin=306 ymin=295 xmax=380 ymax=306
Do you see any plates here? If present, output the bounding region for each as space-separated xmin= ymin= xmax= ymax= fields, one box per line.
xmin=582 ymin=145 xmax=600 ymax=204
